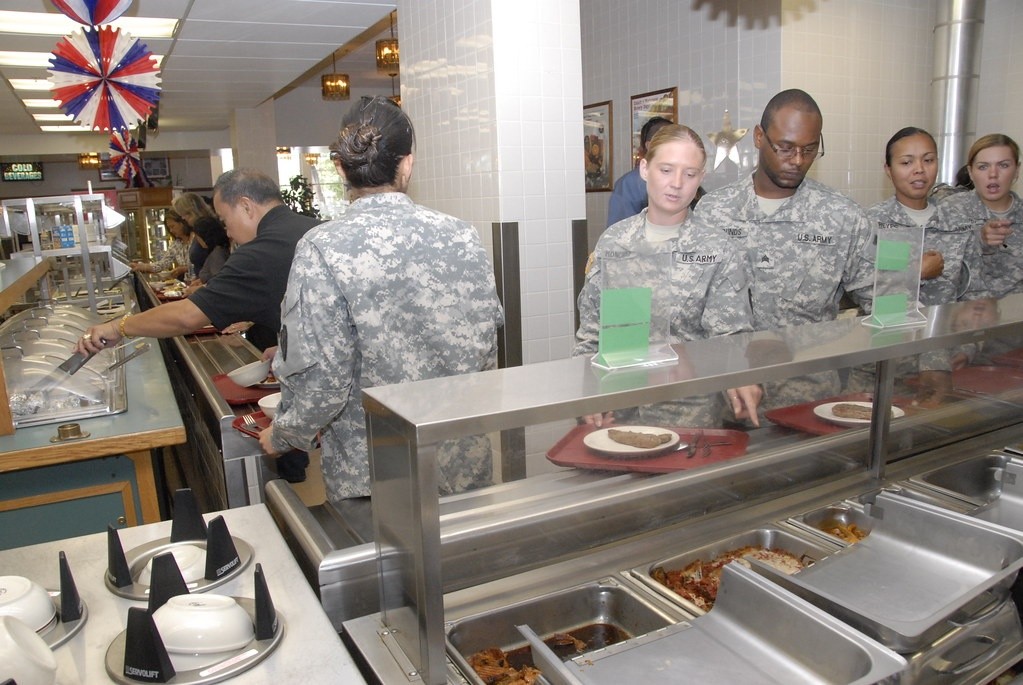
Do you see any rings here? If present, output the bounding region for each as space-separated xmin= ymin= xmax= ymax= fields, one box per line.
xmin=82 ymin=334 xmax=92 ymax=341
xmin=731 ymin=396 xmax=740 ymax=403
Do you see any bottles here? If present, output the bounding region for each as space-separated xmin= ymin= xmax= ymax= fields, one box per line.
xmin=190 ymin=264 xmax=196 ymax=280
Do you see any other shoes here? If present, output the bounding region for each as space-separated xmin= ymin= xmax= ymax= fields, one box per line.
xmin=275 ymin=463 xmax=305 ymax=482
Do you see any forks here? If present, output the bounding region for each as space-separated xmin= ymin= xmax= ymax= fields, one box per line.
xmin=702 ymin=441 xmax=712 ymax=456
xmin=242 ymin=414 xmax=266 ymax=430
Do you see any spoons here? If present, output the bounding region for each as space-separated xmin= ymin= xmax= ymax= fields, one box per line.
xmin=671 ymin=440 xmax=735 ymax=451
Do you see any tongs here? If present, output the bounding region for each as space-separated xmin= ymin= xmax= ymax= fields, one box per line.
xmin=102 ymin=342 xmax=152 ymax=376
xmin=27 ymin=338 xmax=107 ymax=396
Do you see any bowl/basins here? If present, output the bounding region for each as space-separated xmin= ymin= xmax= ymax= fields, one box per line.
xmin=258 ymin=391 xmax=281 ymax=420
xmin=227 ymin=358 xmax=271 ymax=388
xmin=137 ymin=544 xmax=207 ymax=586
xmin=152 ymin=593 xmax=256 ymax=672
xmin=0 ymin=575 xmax=58 ymax=685
xmin=150 ymin=282 xmax=166 ymax=290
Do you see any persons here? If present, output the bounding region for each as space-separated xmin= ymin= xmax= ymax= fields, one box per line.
xmin=573 ymin=124 xmax=769 ymax=427
xmin=258 ymin=95 xmax=503 ymax=502
xmin=130 ymin=192 xmax=278 ymax=351
xmin=928 ymin=134 xmax=1023 ymax=364
xmin=606 ymin=117 xmax=674 ymax=227
xmin=844 ymin=127 xmax=988 ymax=395
xmin=694 ymin=87 xmax=918 ymax=431
xmin=70 ymin=168 xmax=322 ymax=483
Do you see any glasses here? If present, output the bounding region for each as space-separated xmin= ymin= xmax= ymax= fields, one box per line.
xmin=761 ymin=123 xmax=825 ymax=161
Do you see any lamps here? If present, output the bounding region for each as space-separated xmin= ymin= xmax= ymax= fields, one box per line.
xmin=375 ymin=12 xmax=400 ymax=73
xmin=303 ymin=153 xmax=320 ymax=167
xmin=78 ymin=152 xmax=103 ymax=170
xmin=388 ymin=73 xmax=400 ymax=108
xmin=138 ymin=118 xmax=146 ymax=150
xmin=321 ymin=51 xmax=350 ymax=101
xmin=148 ymin=91 xmax=160 ymax=131
xmin=276 ymin=146 xmax=291 ymax=159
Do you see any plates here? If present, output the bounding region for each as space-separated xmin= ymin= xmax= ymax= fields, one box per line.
xmin=257 ymin=380 xmax=280 ymax=389
xmin=164 ymin=291 xmax=182 ymax=297
xmin=582 ymin=425 xmax=681 ymax=457
xmin=812 ymin=400 xmax=905 ymax=428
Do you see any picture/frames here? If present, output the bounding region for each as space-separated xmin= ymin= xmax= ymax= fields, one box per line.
xmin=142 ymin=158 xmax=171 ymax=179
xmin=631 ymin=87 xmax=678 ymax=170
xmin=98 ymin=160 xmax=125 ymax=181
xmin=583 ymin=100 xmax=613 ymax=192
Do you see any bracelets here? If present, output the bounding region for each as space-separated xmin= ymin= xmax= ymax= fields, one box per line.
xmin=119 ymin=312 xmax=136 ymax=340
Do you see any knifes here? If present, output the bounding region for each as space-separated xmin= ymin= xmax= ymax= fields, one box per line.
xmin=241 ymin=424 xmax=259 ymax=434
xmin=686 ymin=430 xmax=699 ymax=457
